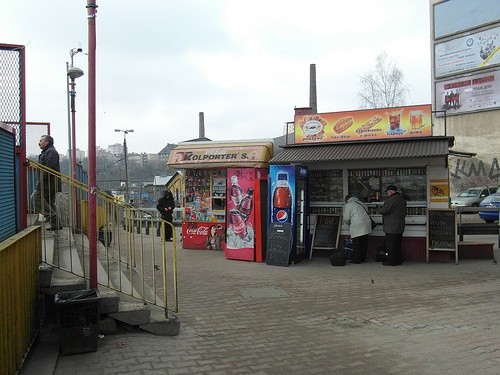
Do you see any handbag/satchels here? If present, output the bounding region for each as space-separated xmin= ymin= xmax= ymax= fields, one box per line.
xmin=353 ymin=201 xmax=376 ymax=230
xmin=329 ymin=248 xmax=346 ymax=266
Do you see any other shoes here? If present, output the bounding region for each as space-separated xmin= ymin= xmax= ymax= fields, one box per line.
xmin=45 ymin=227 xmax=63 ymax=231
xmin=349 ymin=260 xmax=360 ymax=264
xmin=383 ymin=262 xmax=395 ymax=265
xmin=45 ymin=214 xmax=56 ymax=222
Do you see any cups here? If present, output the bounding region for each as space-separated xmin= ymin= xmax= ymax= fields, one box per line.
xmin=410 ymin=111 xmax=428 ymax=131
xmin=389 ymin=114 xmax=401 ymax=131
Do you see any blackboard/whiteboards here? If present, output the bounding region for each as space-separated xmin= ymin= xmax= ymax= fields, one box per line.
xmin=266 ymin=223 xmax=292 ymax=267
xmin=427 ymin=208 xmax=457 ymax=251
xmin=311 ymin=214 xmax=342 ymax=249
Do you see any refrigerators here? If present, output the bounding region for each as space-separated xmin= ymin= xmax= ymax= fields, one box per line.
xmin=267 ymin=165 xmax=309 ymax=264
xmin=223 ymin=168 xmax=269 ymax=262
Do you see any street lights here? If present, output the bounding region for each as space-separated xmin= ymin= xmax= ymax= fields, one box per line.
xmin=115 ymin=128 xmax=133 ymax=209
xmin=64 ymin=46 xmax=84 ymax=234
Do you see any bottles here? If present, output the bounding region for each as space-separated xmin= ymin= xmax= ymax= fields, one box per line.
xmin=214 ymin=224 xmax=221 ymax=231
xmin=228 ymin=176 xmax=254 ymax=242
xmin=272 ymin=173 xmax=293 ymax=223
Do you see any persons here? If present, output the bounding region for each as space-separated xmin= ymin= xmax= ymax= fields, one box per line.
xmin=129 ymin=199 xmax=135 ymax=215
xmin=445 ymin=90 xmax=459 ymax=110
xmin=379 ymin=185 xmax=407 ymax=266
xmin=156 ymin=189 xmax=175 ymax=242
xmin=343 ymin=195 xmax=371 ymax=264
xmin=103 ymin=190 xmax=119 ymax=224
xmin=30 ymin=135 xmax=63 ymax=231
xmin=205 ymin=224 xmax=225 ymax=250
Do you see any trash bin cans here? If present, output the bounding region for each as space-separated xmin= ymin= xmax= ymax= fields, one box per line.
xmin=99 ymin=223 xmax=112 ymax=246
xmin=54 ymin=289 xmax=102 ymax=355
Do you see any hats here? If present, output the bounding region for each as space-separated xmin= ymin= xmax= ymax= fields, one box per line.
xmin=384 ymin=185 xmax=397 ymax=192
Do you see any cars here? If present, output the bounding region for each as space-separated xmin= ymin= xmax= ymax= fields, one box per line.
xmin=450 ymin=187 xmax=499 ymax=214
xmin=480 ymin=193 xmax=500 ymax=223
